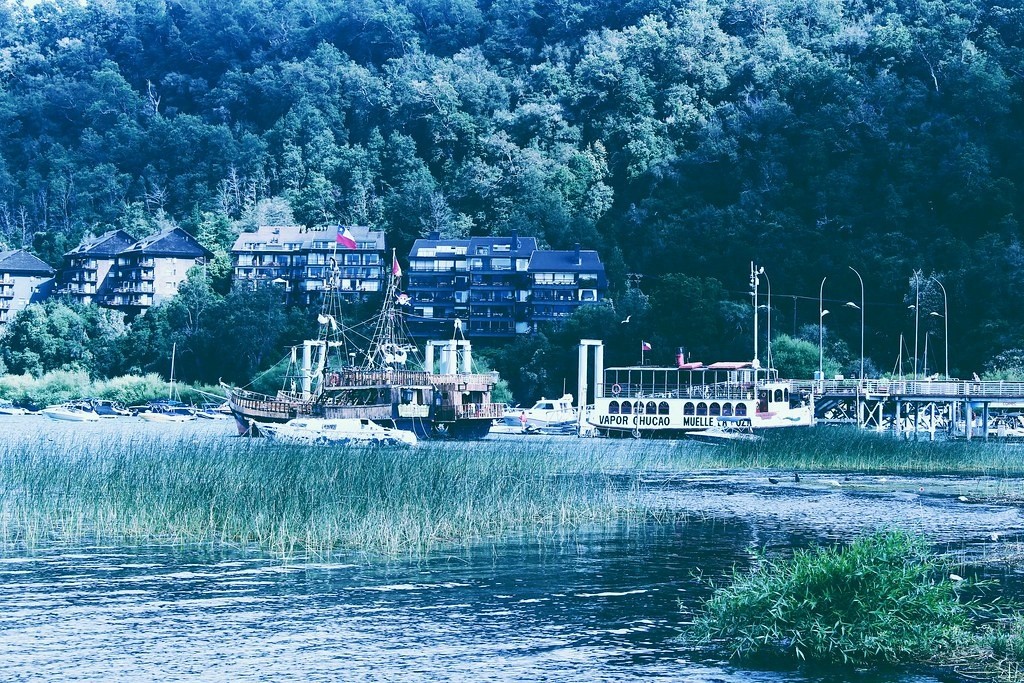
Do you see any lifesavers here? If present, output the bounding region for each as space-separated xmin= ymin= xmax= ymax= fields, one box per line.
xmin=611 ymin=383 xmax=622 ymax=394
xmin=330 ymin=375 xmax=338 ymax=386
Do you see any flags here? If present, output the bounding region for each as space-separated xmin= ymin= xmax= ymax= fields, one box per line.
xmin=393 ymin=256 xmax=402 ymax=276
xmin=337 ymin=224 xmax=356 ymax=249
xmin=643 ymin=342 xmax=651 ymax=351
xmin=395 ymin=289 xmax=416 ymax=307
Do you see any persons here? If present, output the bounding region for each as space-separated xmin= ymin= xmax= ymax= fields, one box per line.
xmin=931 ymin=373 xmax=939 ymax=381
xmin=972 ymin=373 xmax=981 ymax=395
xmin=519 ymin=411 xmax=527 ymax=429
xmin=864 ymin=374 xmax=868 ymax=379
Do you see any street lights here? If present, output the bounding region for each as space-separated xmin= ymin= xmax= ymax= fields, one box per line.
xmin=930 ymin=311 xmax=948 ymax=380
xmin=907 ymin=304 xmax=919 ymax=395
xmin=820 ymin=310 xmax=830 ymax=391
xmin=846 ymin=302 xmax=864 ymax=393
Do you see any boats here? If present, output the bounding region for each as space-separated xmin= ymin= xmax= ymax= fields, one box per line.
xmin=244 ymin=416 xmax=418 ymax=448
xmin=0 ymin=396 xmax=132 ymax=422
xmin=503 ymin=408 xmax=528 ymax=426
xmin=219 ymin=220 xmax=508 ymax=440
xmin=685 ymin=417 xmax=759 ymax=443
xmin=523 ymin=378 xmax=578 ymax=434
xmin=587 ymin=340 xmax=814 ymax=440
xmin=189 ymin=403 xmax=238 ymax=421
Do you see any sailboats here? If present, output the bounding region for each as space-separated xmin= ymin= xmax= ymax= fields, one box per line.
xmin=137 ymin=342 xmax=197 ymax=422
